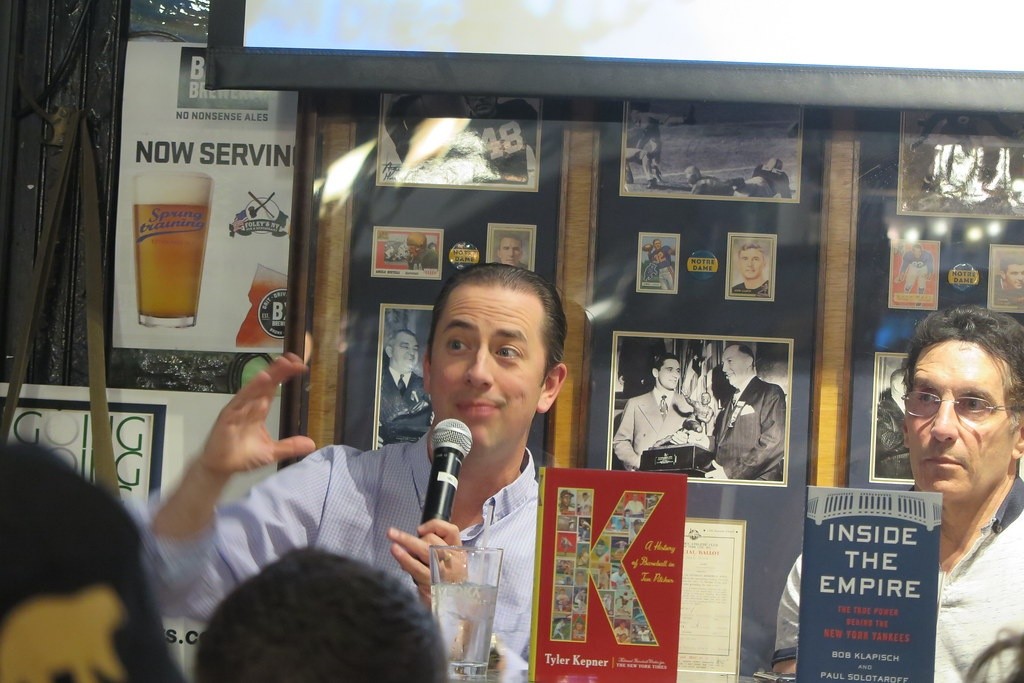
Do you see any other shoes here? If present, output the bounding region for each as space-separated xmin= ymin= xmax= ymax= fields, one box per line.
xmin=624 ymin=176 xmax=634 ymax=184
xmin=647 ymin=178 xmax=658 ymax=189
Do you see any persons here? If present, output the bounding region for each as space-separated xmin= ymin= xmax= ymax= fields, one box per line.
xmin=0 ymin=440 xmax=184 ymax=682
xmin=498 ymin=236 xmax=526 ymax=268
xmin=450 ymin=94 xmax=536 ymax=184
xmin=995 ymin=257 xmax=1024 ymax=300
xmin=732 ymin=242 xmax=769 ymax=293
xmin=127 ymin=263 xmax=569 ymax=683
xmin=626 ymin=110 xmax=792 ymax=198
xmin=771 ymin=307 xmax=1024 ymax=683
xmin=407 ymin=232 xmax=438 ymax=269
xmin=192 ymin=548 xmax=446 ymax=683
xmin=648 ymin=239 xmax=676 ymax=289
xmin=613 ymin=344 xmax=782 ymax=482
xmin=875 ymin=369 xmax=910 ymax=477
xmin=552 ymin=483 xmax=658 ymax=642
xmin=379 ymin=328 xmax=427 ymax=440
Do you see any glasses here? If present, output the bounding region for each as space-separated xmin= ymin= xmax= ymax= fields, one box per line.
xmin=900 ymin=390 xmax=1023 ymax=421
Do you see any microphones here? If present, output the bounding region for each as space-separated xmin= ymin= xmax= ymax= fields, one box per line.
xmin=412 ymin=418 xmax=473 ymax=584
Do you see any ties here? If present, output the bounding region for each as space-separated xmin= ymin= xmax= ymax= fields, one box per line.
xmin=659 ymin=395 xmax=669 ymax=421
xmin=397 ymin=374 xmax=407 ymax=397
xmin=725 ymin=391 xmax=741 ymax=428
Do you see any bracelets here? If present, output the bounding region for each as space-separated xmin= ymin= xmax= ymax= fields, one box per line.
xmin=488 ymin=633 xmax=499 ymax=670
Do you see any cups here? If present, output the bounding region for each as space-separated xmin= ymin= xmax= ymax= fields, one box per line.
xmin=936 ymin=571 xmax=945 ymax=628
xmin=428 ymin=544 xmax=504 ymax=682
xmin=130 ymin=171 xmax=214 ymax=330
